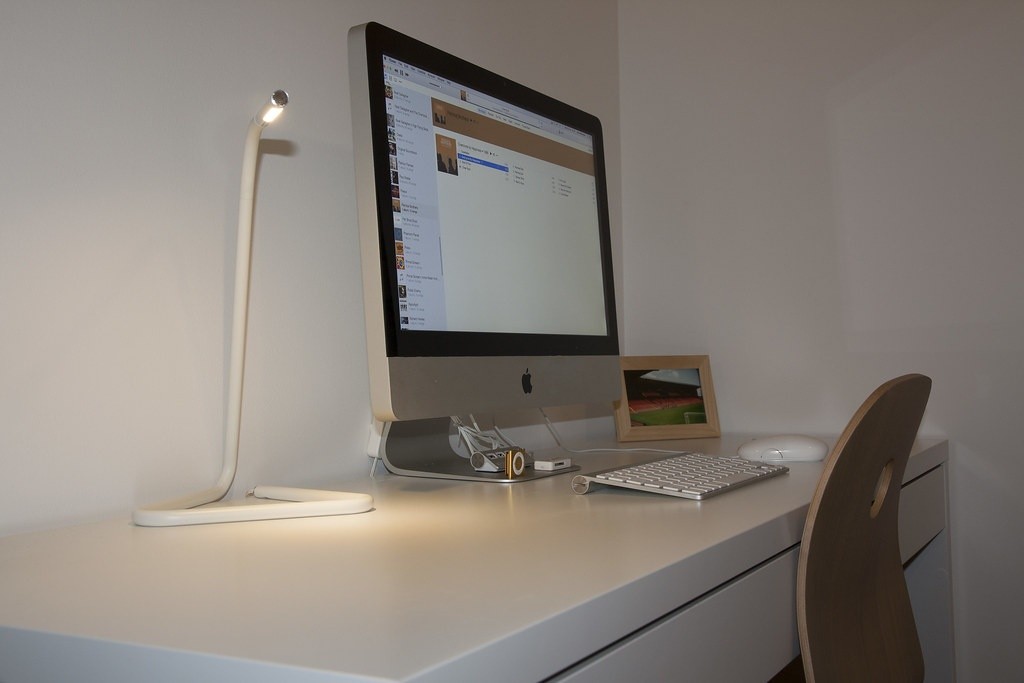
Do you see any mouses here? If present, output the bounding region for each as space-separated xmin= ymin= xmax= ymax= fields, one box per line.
xmin=736 ymin=435 xmax=829 ymax=462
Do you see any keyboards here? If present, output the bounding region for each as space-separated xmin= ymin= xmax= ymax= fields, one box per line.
xmin=582 ymin=451 xmax=789 ymax=501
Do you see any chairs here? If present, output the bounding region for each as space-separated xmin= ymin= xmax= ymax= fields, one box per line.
xmin=795 ymin=373 xmax=932 ymax=683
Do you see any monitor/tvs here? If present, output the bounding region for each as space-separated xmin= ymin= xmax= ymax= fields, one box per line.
xmin=349 ymin=22 xmax=624 ymax=482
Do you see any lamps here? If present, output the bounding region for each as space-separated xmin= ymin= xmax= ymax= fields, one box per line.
xmin=132 ymin=90 xmax=374 ymax=527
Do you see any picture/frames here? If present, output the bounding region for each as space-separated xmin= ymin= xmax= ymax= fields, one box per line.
xmin=613 ymin=355 xmax=722 ymax=443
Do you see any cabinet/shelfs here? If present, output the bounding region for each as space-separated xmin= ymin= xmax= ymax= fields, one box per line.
xmin=0 ymin=429 xmax=957 ymax=683
